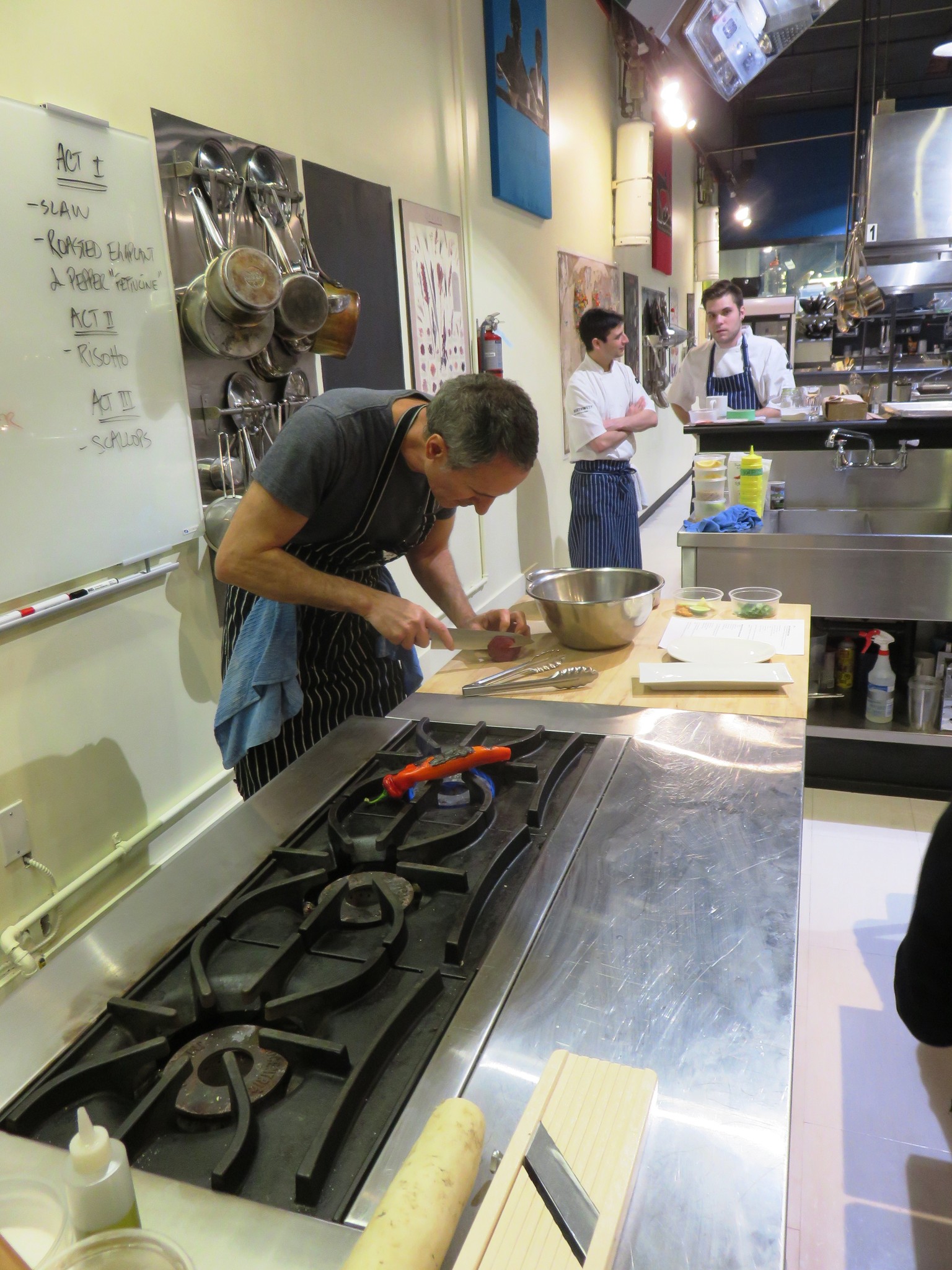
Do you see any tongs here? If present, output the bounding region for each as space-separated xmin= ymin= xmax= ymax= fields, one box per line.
xmin=462 ymin=650 xmax=600 ymax=697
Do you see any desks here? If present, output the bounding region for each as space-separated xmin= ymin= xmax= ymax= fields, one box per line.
xmin=415 ymin=593 xmax=812 ymax=718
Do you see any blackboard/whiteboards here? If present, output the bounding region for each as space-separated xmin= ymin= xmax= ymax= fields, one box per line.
xmin=0 ymin=95 xmax=206 ymax=631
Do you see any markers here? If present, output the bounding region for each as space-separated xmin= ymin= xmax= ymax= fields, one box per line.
xmin=20 ymin=593 xmax=71 ymax=617
xmin=70 ymin=577 xmax=119 ymax=600
xmin=0 ymin=610 xmax=22 ymax=626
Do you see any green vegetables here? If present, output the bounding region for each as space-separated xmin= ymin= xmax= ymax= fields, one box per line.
xmin=735 ymin=602 xmax=774 ymax=619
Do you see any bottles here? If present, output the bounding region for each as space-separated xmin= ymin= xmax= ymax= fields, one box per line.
xmin=780 ymin=388 xmax=803 ymax=408
xmin=64 ymin=1107 xmax=141 ymax=1242
xmin=739 ymin=445 xmax=762 ymax=519
xmin=764 ymin=255 xmax=787 ymax=296
xmin=835 ymin=635 xmax=854 ymax=689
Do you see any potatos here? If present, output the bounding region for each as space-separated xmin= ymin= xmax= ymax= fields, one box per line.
xmin=487 ymin=635 xmax=521 ymax=662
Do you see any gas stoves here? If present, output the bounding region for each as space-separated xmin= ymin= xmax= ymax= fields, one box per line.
xmin=0 ymin=716 xmax=633 ymax=1231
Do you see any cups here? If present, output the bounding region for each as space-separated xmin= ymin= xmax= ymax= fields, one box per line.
xmin=908 ymin=656 xmax=942 ymax=731
xmin=808 ymin=629 xmax=835 ymax=693
xmin=801 ymin=385 xmax=821 ymax=420
xmin=706 ymin=395 xmax=728 ymax=416
xmin=894 ymin=382 xmax=912 ymax=402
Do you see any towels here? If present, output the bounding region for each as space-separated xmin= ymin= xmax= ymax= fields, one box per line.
xmin=683 ymin=505 xmax=764 ymax=534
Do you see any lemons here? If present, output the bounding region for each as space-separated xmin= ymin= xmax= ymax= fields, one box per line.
xmin=689 ymin=606 xmax=710 ymax=614
xmin=697 ymin=597 xmax=707 ymax=606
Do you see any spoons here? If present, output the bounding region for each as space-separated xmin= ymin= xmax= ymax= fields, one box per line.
xmin=867 ymin=373 xmax=881 ymax=403
xmin=844 ymin=357 xmax=855 ymax=369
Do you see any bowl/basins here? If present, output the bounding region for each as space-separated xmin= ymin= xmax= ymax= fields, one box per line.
xmin=916 ymin=385 xmax=951 ymax=395
xmin=688 ymin=407 xmax=812 ymax=424
xmin=33 ymin=1228 xmax=195 ymax=1270
xmin=526 ymin=568 xmax=783 ymax=653
xmin=691 ymin=454 xmax=728 ymax=523
xmin=526 ymin=567 xmax=586 ymax=582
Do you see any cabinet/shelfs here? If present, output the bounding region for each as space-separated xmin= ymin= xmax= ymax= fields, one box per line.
xmin=830 ymin=315 xmax=952 ymax=358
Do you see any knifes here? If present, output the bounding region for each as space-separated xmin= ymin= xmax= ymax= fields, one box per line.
xmin=427 ymin=628 xmax=535 ymax=650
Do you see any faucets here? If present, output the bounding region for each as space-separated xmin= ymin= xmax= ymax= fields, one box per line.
xmin=824 ymin=427 xmax=920 ymax=472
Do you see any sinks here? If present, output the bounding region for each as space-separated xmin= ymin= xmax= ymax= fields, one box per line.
xmin=866 ymin=508 xmax=952 ymax=623
xmin=758 ymin=507 xmax=874 ymax=617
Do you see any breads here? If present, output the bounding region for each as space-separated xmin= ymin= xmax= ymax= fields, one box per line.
xmin=340 ymin=1097 xmax=486 ymax=1269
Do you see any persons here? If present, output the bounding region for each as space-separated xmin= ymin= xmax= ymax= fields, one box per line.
xmin=212 ymin=373 xmax=539 ymax=801
xmin=564 ymin=307 xmax=658 ymax=570
xmin=894 ymin=802 xmax=952 ymax=1049
xmin=665 ymin=279 xmax=795 ymax=439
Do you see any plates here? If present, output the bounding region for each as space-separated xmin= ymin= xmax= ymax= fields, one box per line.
xmin=637 ymin=636 xmax=795 ymax=691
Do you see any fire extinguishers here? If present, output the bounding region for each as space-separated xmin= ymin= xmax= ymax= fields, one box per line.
xmin=478 ymin=312 xmax=503 ymax=379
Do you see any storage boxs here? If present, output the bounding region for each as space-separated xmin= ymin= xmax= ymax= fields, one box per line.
xmin=822 ymin=394 xmax=867 ymax=421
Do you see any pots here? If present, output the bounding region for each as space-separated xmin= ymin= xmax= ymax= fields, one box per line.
xmin=834 ymin=219 xmax=885 ymax=318
xmin=178 ymin=168 xmax=361 ymax=362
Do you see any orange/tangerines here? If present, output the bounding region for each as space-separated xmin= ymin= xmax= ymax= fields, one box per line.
xmin=695 ymin=460 xmax=722 ymax=468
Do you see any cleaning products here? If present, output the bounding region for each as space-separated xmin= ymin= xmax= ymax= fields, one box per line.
xmin=858 ymin=628 xmax=896 ymax=723
